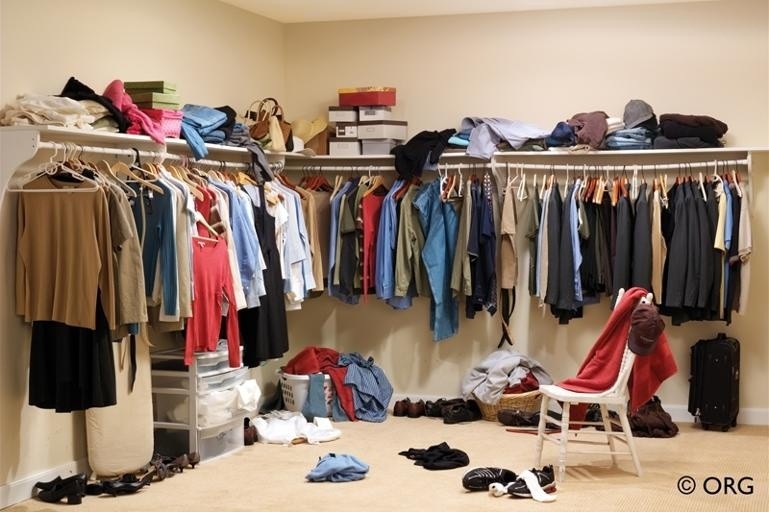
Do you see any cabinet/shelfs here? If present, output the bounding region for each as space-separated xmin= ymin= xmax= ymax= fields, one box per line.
xmin=150 ymin=351 xmax=248 ymax=463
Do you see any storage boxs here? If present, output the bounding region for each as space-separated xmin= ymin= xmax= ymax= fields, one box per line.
xmin=328 ymin=86 xmax=408 ymax=155
xmin=123 ymin=80 xmax=184 ymax=139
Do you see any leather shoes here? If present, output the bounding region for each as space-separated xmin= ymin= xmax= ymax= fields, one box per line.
xmin=244 ymin=418 xmax=258 ymax=445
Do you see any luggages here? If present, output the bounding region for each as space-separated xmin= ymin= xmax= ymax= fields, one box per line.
xmin=688 ymin=333 xmax=739 ymax=432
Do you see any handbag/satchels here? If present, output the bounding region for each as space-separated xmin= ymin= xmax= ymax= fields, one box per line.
xmin=235 ymin=99 xmax=293 ymax=153
xmin=629 ymin=396 xmax=679 ymax=438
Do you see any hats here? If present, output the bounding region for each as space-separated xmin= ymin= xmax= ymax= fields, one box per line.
xmin=624 ymin=99 xmax=653 ymax=129
xmin=629 ymin=305 xmax=666 ymax=356
xmin=291 ymin=118 xmax=327 ymax=145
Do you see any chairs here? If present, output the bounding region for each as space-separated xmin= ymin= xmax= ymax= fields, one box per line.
xmin=535 ymin=287 xmax=654 ymax=481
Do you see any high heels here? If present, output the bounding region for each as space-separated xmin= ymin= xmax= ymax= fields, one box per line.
xmin=35 ymin=452 xmax=200 ymax=505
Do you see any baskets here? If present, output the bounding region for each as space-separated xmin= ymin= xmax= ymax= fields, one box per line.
xmin=472 ymin=389 xmax=543 ymax=422
xmin=277 ymin=368 xmax=334 ymax=418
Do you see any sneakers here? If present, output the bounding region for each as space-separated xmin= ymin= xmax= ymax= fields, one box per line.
xmin=463 ymin=466 xmax=515 ymax=491
xmin=393 ymin=398 xmax=482 ymax=424
xmin=497 ymin=409 xmax=536 ymax=427
xmin=507 ymin=468 xmax=556 ymax=498
